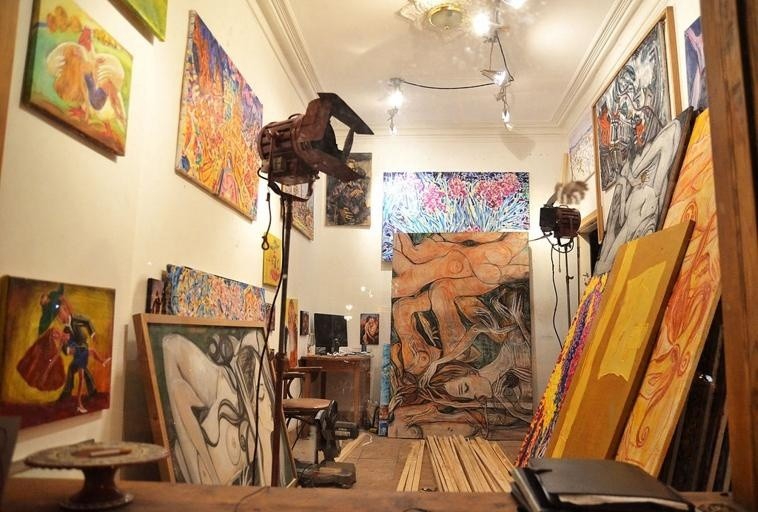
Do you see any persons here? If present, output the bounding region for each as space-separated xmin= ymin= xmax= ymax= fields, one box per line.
xmin=38 ymin=284 xmax=98 ymax=403
xmin=161 ymin=330 xmax=274 ymax=487
xmin=391 ymin=232 xmax=533 ymax=439
xmin=16 ymin=328 xmax=112 ymax=413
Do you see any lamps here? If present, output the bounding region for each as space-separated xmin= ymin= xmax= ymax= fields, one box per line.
xmin=399 ymin=0 xmax=477 ymax=45
xmin=247 ymin=87 xmax=375 ymax=489
xmin=533 ymin=201 xmax=583 ymax=353
xmin=380 ymin=0 xmax=517 ymax=135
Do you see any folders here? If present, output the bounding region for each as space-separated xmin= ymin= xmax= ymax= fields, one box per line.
xmin=510 ymin=458 xmax=696 ymax=512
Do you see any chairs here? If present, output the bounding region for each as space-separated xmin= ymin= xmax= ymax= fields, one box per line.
xmin=270 ymin=346 xmax=341 ymax=468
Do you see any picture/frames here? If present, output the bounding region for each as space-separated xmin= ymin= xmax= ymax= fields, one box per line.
xmin=589 ymin=3 xmax=682 ymax=247
xmin=132 ymin=309 xmax=301 ymax=493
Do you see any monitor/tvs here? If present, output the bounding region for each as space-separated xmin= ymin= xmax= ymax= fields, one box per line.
xmin=314 ymin=313 xmax=347 ymax=355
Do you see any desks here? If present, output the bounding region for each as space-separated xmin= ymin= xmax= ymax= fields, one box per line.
xmin=301 ymin=353 xmax=374 ymax=437
